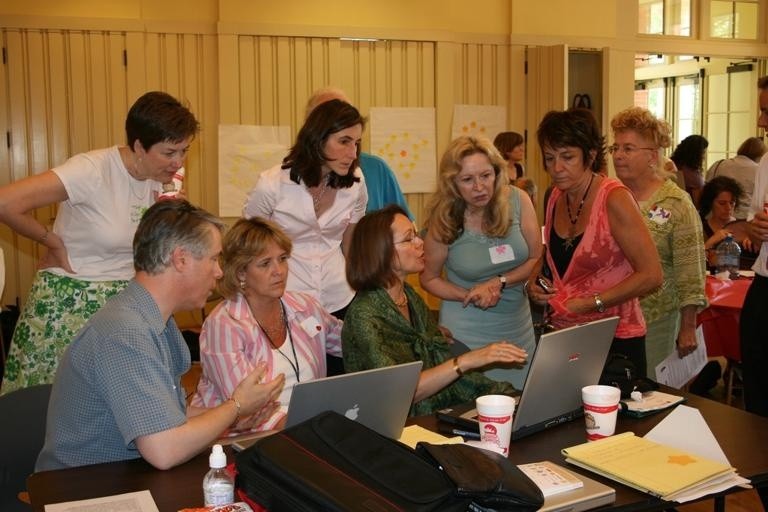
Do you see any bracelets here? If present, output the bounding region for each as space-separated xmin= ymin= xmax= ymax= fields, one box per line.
xmin=39 ymin=229 xmax=49 ymax=244
xmin=225 ymin=396 xmax=241 ymax=429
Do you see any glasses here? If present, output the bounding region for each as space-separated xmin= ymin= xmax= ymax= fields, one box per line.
xmin=608 ymin=145 xmax=653 ymax=155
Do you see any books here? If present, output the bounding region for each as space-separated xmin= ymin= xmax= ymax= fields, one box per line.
xmin=517 ymin=463 xmax=584 ymax=498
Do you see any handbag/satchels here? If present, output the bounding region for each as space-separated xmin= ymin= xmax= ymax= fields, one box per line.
xmin=599 ymin=353 xmax=658 ymax=397
xmin=236 ymin=409 xmax=473 ymax=512
xmin=690 ymin=159 xmax=723 ymax=210
xmin=415 ymin=442 xmax=543 ymax=512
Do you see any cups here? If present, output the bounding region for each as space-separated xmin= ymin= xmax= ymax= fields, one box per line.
xmin=581 ymin=385 xmax=623 ymax=442
xmin=475 ymin=394 xmax=514 ymax=457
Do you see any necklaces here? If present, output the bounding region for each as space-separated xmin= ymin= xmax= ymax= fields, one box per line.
xmin=247 ymin=297 xmax=284 ymax=340
xmin=125 ymin=146 xmax=150 ymax=200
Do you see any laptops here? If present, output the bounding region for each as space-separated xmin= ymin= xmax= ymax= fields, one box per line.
xmin=434 ymin=316 xmax=620 ymax=443
xmin=233 ymin=362 xmax=423 ymax=454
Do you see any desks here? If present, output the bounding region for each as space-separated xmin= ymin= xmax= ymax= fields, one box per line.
xmin=698 ymin=269 xmax=754 ymax=362
xmin=24 ymin=376 xmax=768 ymax=511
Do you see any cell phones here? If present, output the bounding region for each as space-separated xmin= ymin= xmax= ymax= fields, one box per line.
xmin=536 ymin=276 xmax=554 ymax=294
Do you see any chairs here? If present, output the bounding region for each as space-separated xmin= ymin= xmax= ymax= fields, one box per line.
xmin=0 ymin=384 xmax=52 ymax=511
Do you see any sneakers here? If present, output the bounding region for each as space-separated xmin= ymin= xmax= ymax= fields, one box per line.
xmin=688 ymin=360 xmax=721 ymax=396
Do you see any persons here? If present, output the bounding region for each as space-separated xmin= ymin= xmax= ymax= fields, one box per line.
xmin=33 ymin=197 xmax=286 ymax=475
xmin=189 ymin=216 xmax=346 ymax=440
xmin=241 ymin=98 xmax=369 ymax=379
xmin=0 ymin=90 xmax=204 ymax=400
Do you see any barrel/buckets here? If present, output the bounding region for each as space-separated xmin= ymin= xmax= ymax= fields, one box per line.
xmin=716 ymin=233 xmax=743 ymax=279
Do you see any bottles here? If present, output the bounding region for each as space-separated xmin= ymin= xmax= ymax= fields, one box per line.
xmin=202 ymin=444 xmax=235 ymax=508
